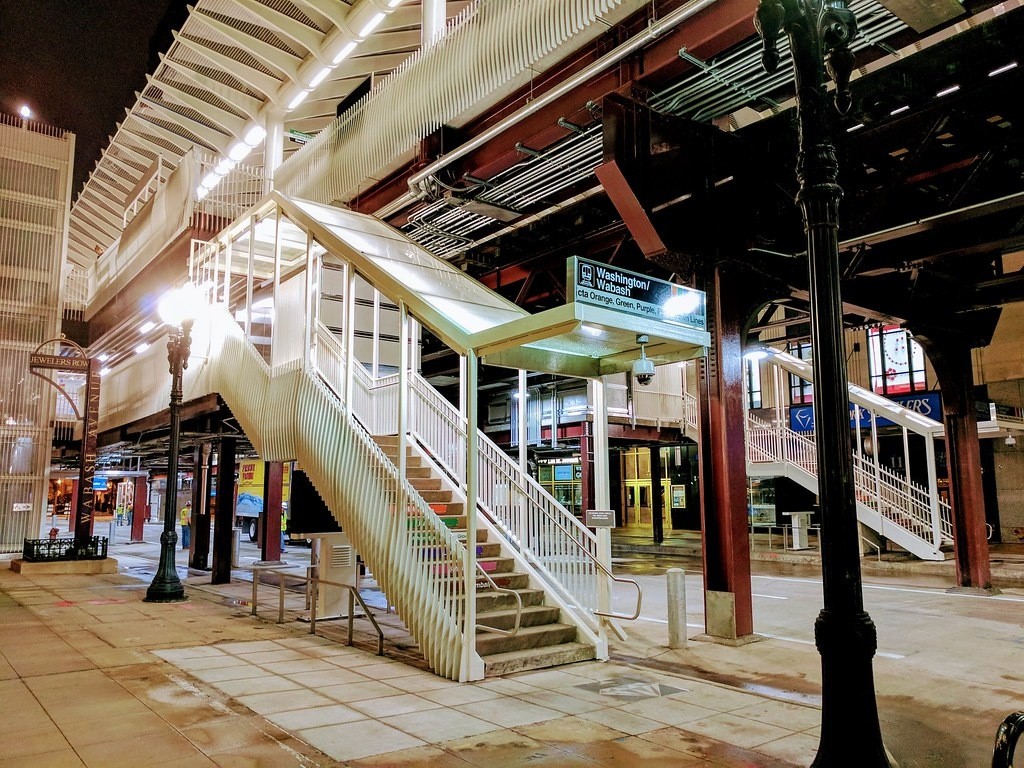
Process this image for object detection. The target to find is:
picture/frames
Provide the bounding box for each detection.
[670,485,686,509]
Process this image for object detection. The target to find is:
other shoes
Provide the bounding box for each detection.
[183,545,190,549]
[280,549,288,554]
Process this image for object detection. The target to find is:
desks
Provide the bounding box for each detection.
[781,511,816,550]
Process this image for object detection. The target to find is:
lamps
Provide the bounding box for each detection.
[742,344,768,361]
[194,0,404,202]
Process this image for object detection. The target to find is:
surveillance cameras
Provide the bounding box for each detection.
[632,360,656,386]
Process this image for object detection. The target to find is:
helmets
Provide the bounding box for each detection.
[186,501,192,506]
[128,501,132,505]
[118,503,123,507]
[282,502,288,509]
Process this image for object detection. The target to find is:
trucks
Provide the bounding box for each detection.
[234,456,315,550]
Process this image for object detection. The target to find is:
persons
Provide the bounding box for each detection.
[179,501,192,550]
[281,502,287,553]
[116,503,123,526]
[125,501,133,526]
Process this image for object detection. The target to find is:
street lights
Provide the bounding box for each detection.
[145,286,202,603]
